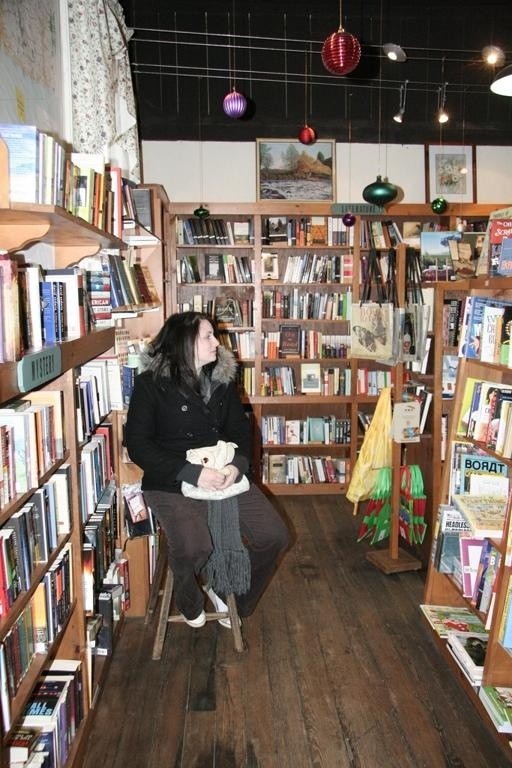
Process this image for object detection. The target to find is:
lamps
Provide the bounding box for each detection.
[392,82,408,123]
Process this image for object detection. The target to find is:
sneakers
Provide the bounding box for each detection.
[178,604,207,629]
[203,582,242,630]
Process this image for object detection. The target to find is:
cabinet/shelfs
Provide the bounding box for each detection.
[349,204,512,768]
[2,181,168,766]
[162,201,353,496]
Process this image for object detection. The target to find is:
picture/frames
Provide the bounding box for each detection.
[255,137,337,202]
[424,140,477,202]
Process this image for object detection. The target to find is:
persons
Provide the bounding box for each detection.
[126,313,290,631]
[126,311,290,629]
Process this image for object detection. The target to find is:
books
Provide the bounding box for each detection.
[175,216,255,328]
[261,449,351,484]
[4,337,152,768]
[261,286,352,359]
[261,361,352,446]
[4,252,160,362]
[261,216,354,284]
[4,126,152,241]
[359,219,418,365]
[215,331,255,434]
[420,207,510,733]
[358,362,417,444]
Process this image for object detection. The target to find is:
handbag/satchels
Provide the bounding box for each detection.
[345,386,394,516]
[351,247,430,365]
[181,439,250,501]
[356,464,429,549]
